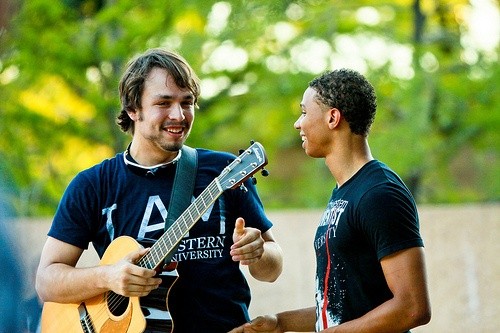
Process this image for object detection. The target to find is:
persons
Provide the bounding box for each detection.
[36,47,283,333]
[225,67,432,333]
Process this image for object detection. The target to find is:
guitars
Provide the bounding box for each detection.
[40,139,269,333]
